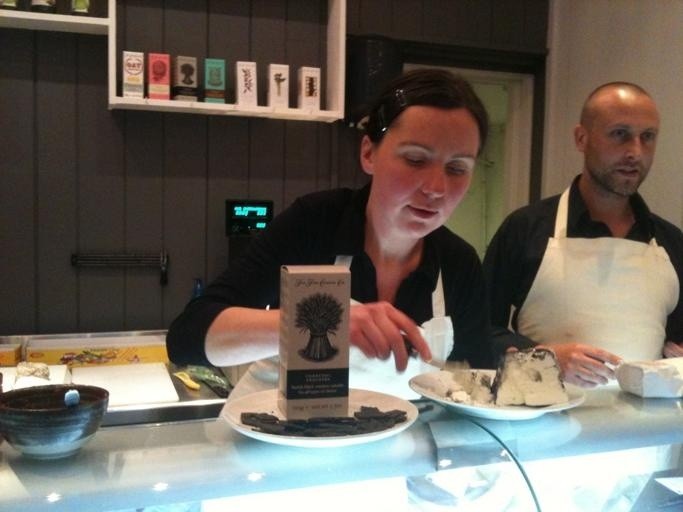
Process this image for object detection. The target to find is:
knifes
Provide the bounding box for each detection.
[165,361,227,396]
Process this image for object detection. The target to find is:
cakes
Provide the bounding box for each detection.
[439,347,567,405]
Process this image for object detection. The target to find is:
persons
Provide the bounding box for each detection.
[165,68,483,368]
[455,80,683,391]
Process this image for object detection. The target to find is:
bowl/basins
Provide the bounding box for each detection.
[1,382,110,461]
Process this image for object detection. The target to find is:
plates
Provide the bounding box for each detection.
[220,386,420,450]
[406,367,587,422]
